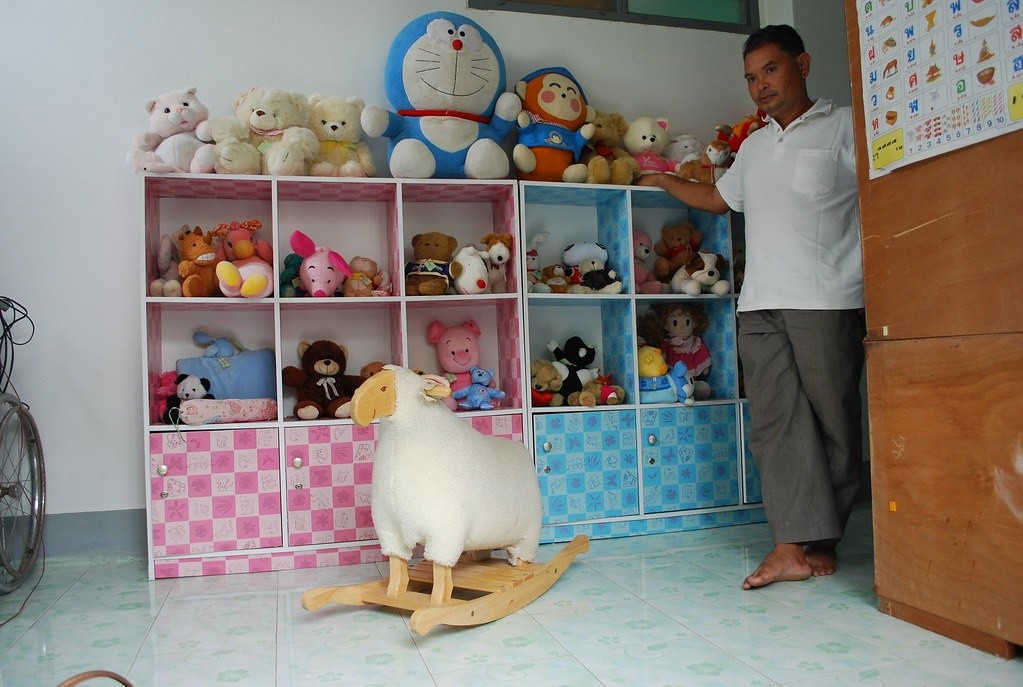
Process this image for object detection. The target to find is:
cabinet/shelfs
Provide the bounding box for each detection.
[139,168,772,584]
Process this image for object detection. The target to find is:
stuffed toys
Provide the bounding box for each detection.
[282,339,389,420]
[405,232,514,295]
[511,65,767,184]
[530,335,625,407]
[307,92,377,178]
[126,85,217,173]
[279,229,394,298]
[636,301,713,406]
[525,231,623,294]
[211,85,319,175]
[631,222,731,295]
[413,319,507,410]
[149,220,274,299]
[154,332,276,424]
[360,11,523,179]
[335,363,542,564]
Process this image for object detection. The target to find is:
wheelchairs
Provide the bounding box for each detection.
[0,296,46,594]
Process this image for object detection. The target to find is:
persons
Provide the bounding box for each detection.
[636,26,865,590]
